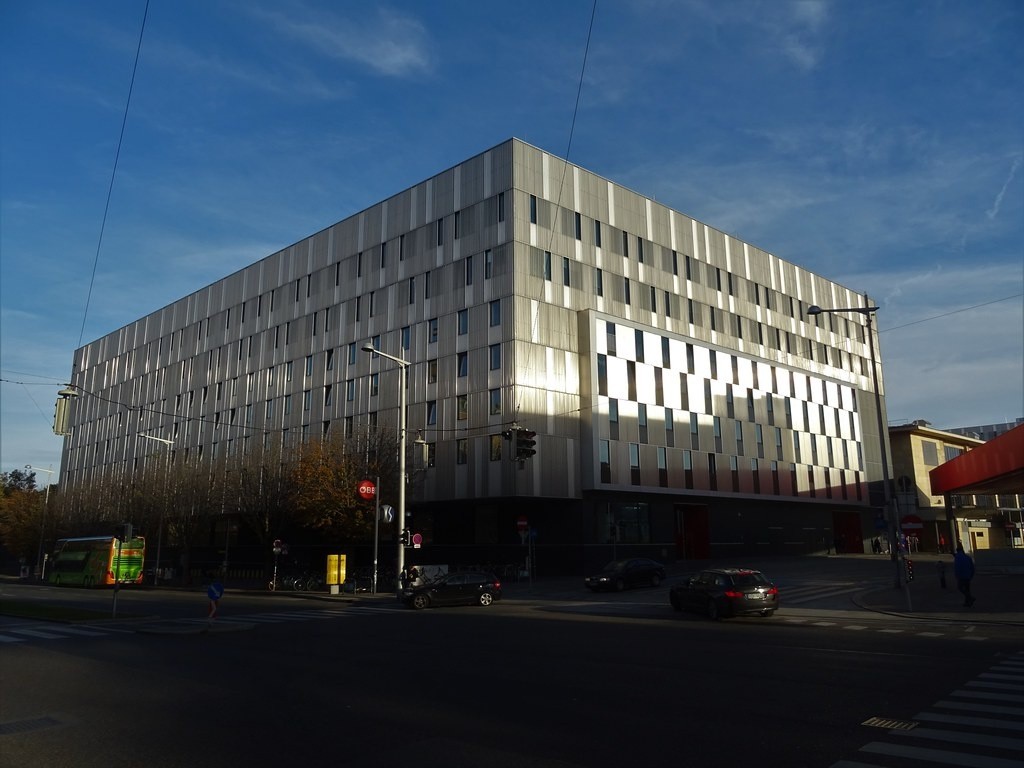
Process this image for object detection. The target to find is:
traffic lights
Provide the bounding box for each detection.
[616,520,624,542]
[40,551,44,560]
[114,523,127,543]
[512,426,537,459]
[904,558,914,584]
[44,553,48,561]
[500,429,513,441]
[413,440,436,472]
[402,530,411,546]
[609,525,617,536]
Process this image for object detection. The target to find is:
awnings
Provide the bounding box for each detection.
[154,566,174,586]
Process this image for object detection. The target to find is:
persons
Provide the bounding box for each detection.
[954,546,976,607]
[403,562,419,588]
[939,534,944,553]
[874,537,882,552]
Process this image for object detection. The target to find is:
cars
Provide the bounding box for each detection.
[400,570,504,611]
[668,568,780,621]
[585,555,669,592]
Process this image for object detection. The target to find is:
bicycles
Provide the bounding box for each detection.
[448,559,524,582]
[263,569,397,593]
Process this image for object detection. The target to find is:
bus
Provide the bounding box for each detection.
[44,534,145,589]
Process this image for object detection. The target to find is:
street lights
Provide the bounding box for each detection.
[360,341,412,600]
[24,463,54,584]
[804,294,912,612]
[137,429,176,586]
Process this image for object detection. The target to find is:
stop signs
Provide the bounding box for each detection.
[517,515,528,529]
[901,514,922,538]
[1004,521,1016,531]
